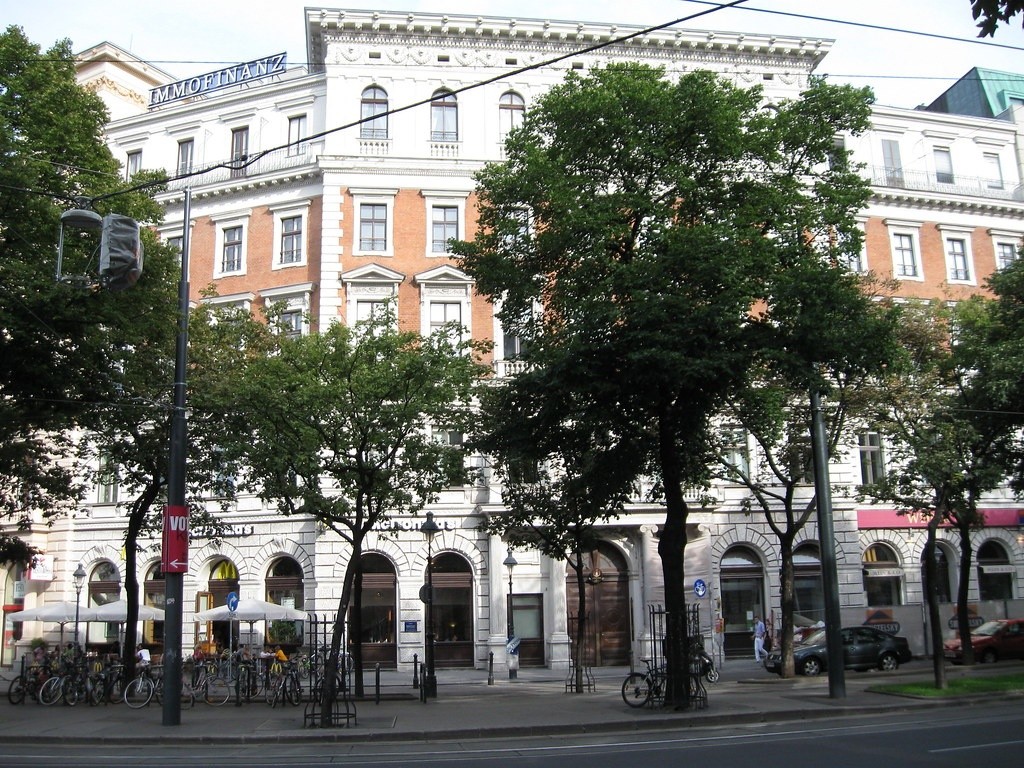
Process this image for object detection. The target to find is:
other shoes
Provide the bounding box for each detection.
[755,661,762,664]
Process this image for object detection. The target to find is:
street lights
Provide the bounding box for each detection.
[419,512,440,699]
[502,551,518,679]
[72,561,86,650]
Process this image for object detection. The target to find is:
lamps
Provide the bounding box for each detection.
[374,12,380,20]
[738,34,744,42]
[572,62,584,69]
[763,73,774,81]
[438,55,451,62]
[544,21,550,28]
[477,17,483,25]
[815,40,822,47]
[510,19,517,27]
[408,14,415,21]
[910,527,914,538]
[340,11,346,18]
[505,58,518,66]
[321,9,328,17]
[578,23,584,31]
[368,52,382,59]
[769,36,776,44]
[611,25,618,33]
[800,39,806,47]
[676,30,683,37]
[708,32,716,40]
[442,15,449,23]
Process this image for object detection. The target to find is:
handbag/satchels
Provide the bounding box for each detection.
[763,631,772,653]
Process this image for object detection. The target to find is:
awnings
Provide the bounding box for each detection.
[864,567,904,577]
[980,564,1016,574]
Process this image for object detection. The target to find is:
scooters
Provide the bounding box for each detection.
[690,642,720,683]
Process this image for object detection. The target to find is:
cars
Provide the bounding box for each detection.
[942,617,1024,665]
[762,624,913,678]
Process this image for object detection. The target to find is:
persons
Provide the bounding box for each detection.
[259,646,288,662]
[194,643,231,664]
[108,640,151,668]
[750,616,769,663]
[235,643,257,671]
[30,643,74,674]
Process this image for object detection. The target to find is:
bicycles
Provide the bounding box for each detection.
[621,659,704,709]
[5,643,352,707]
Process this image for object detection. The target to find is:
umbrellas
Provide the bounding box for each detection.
[6,600,88,665]
[64,598,165,660]
[192,598,307,664]
